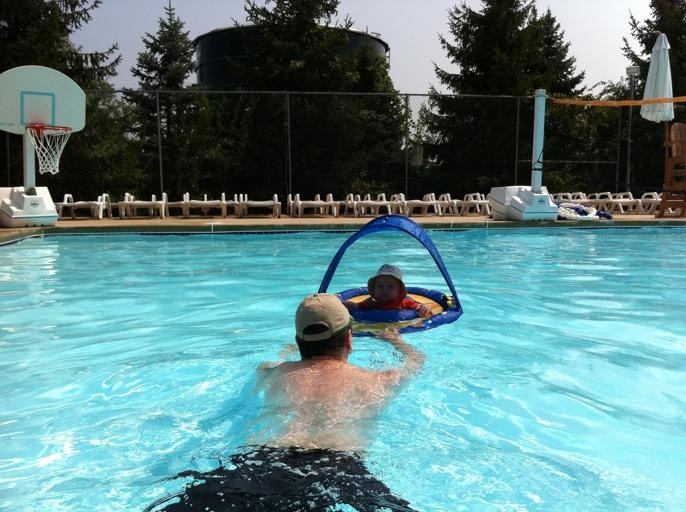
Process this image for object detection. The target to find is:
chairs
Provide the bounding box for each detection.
[56,193,681,219]
[655,123,686,218]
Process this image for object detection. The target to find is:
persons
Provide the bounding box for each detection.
[345,262,436,321]
[169,291,430,511]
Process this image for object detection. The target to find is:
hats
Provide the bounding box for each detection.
[294,293,351,342]
[367,263,408,301]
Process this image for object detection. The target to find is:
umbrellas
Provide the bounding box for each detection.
[639,31,677,124]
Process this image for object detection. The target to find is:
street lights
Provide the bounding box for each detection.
[626,66,641,192]
